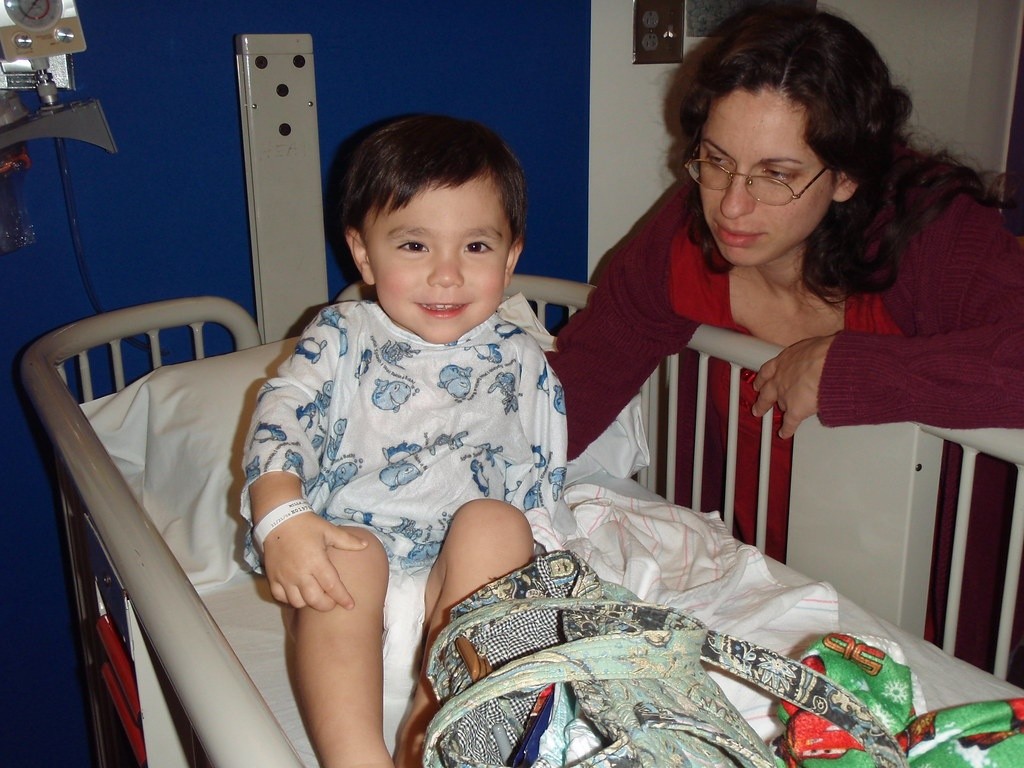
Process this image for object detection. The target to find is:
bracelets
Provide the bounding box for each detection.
[253,499,316,551]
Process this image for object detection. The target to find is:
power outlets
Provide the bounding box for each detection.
[631,0,685,64]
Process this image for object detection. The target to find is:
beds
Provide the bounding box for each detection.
[0,273,1024,768]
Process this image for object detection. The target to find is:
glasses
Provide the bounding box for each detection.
[684,142,829,207]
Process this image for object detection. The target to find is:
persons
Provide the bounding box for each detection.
[544,10,1024,676]
[242,112,568,768]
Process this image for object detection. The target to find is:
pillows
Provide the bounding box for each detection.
[78,335,300,593]
